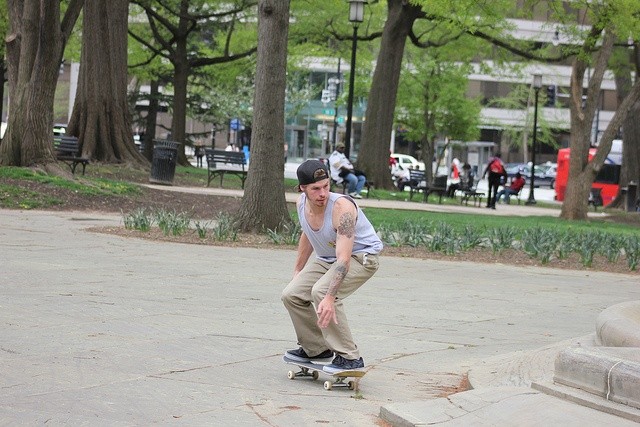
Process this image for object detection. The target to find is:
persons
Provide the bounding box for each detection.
[495,171,526,204]
[482,153,509,208]
[281,158,385,373]
[448,161,473,198]
[327,142,368,198]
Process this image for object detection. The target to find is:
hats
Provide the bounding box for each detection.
[336,143,345,147]
[297,160,329,191]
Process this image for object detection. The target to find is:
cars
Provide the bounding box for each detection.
[390,152,426,182]
[500,161,539,187]
[527,161,557,189]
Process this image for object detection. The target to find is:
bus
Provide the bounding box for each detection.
[554,142,622,207]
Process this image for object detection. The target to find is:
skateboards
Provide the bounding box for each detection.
[283,355,366,390]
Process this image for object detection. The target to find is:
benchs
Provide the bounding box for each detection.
[204,148,249,188]
[504,184,523,204]
[320,156,376,198]
[408,167,447,201]
[458,174,485,207]
[52,135,89,176]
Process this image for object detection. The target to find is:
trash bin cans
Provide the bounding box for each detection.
[149,145,178,186]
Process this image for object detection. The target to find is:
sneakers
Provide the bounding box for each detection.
[285,346,332,363]
[350,192,363,199]
[322,355,364,374]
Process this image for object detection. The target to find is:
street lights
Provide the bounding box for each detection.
[345,0,368,158]
[525,69,543,204]
